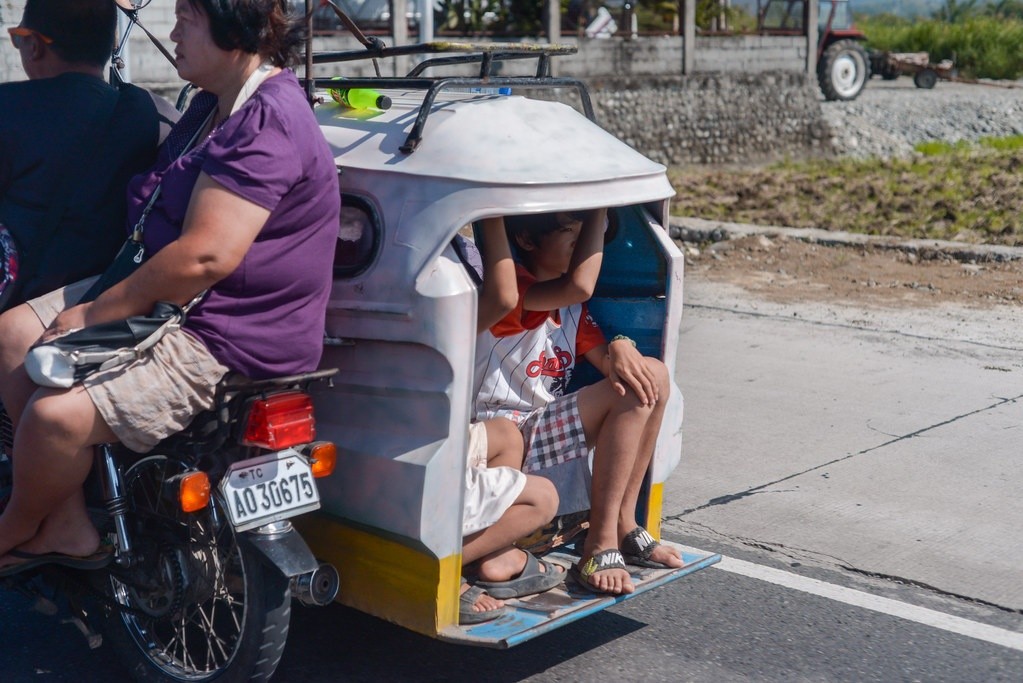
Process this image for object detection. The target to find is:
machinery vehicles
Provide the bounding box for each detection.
[759,1,953,103]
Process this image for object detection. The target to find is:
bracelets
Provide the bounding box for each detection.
[612,335,636,348]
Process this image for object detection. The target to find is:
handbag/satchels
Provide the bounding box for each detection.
[49,238,195,347]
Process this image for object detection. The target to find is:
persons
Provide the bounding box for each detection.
[0,0,336,576]
[458,207,685,625]
[0,0,160,313]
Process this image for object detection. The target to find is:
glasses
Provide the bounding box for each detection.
[7,26,52,49]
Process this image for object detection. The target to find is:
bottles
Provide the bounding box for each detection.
[434,76,515,96]
[325,76,392,111]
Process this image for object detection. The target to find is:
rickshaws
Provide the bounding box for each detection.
[0,1,724,682]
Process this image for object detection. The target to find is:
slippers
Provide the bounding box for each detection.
[0,538,116,578]
[619,526,669,569]
[570,548,628,595]
[459,576,505,624]
[475,547,567,598]
